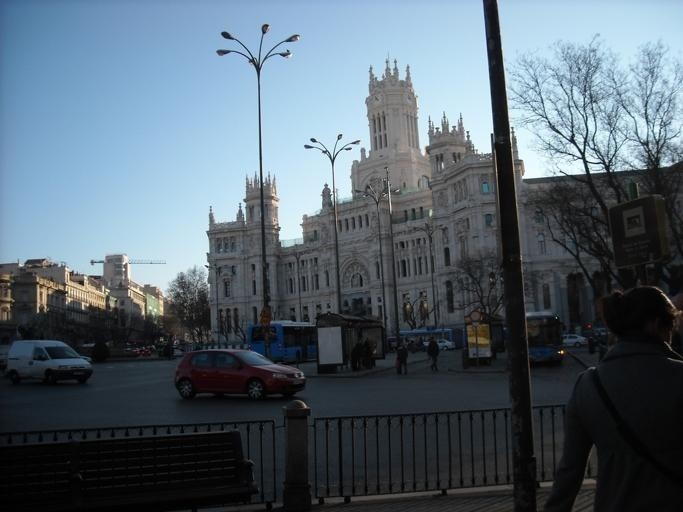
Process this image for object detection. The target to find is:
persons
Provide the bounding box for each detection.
[395,344,409,375]
[540,284,682,511]
[348,336,376,371]
[425,336,440,372]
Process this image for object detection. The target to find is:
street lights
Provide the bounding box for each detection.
[302,133,361,312]
[354,183,400,336]
[412,222,448,325]
[217,24,300,358]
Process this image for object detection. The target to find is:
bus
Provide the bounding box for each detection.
[245,318,318,362]
[525,310,565,367]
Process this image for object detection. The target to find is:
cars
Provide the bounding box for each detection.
[385,326,463,353]
[125,337,169,357]
[173,349,306,401]
[561,326,614,354]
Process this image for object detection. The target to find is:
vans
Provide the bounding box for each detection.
[0,339,95,386]
